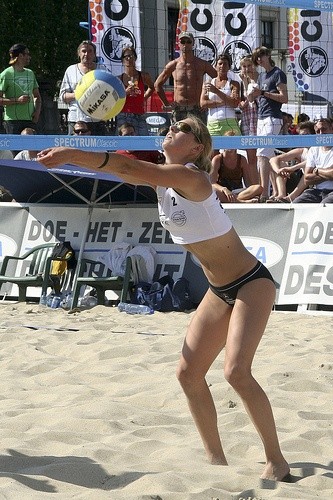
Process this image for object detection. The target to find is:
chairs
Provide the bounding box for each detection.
[70,245,151,310]
[0,243,74,306]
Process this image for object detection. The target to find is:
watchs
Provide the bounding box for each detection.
[314,168,318,174]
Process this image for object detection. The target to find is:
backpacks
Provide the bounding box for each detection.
[149,275,175,310]
[131,281,152,305]
[157,277,192,312]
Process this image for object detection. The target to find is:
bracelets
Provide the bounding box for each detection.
[96,152,109,169]
[260,91,265,96]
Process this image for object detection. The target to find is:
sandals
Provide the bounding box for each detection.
[279,196,292,202]
[266,194,277,202]
[275,196,283,201]
[259,195,268,202]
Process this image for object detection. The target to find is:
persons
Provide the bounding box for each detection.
[156,128,169,164]
[115,124,157,164]
[36,116,290,481]
[209,131,263,203]
[115,46,155,136]
[269,110,333,203]
[61,41,108,137]
[0,43,41,158]
[200,54,242,136]
[13,128,43,161]
[237,56,258,136]
[154,31,218,127]
[73,121,90,136]
[248,46,288,202]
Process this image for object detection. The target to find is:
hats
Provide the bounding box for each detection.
[179,32,194,42]
[9,44,27,64]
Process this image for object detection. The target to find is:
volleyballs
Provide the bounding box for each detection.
[75,69,128,122]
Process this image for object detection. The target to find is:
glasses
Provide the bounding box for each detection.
[255,52,268,60]
[124,132,135,136]
[172,122,202,144]
[179,40,192,44]
[316,126,331,133]
[240,53,252,58]
[74,129,90,133]
[123,55,136,59]
